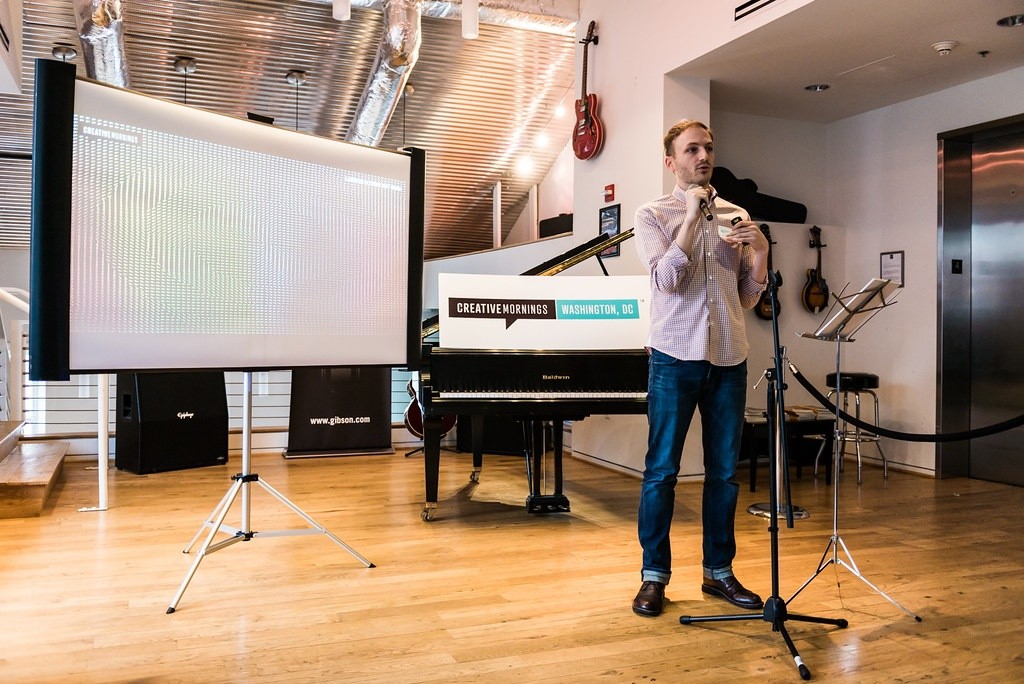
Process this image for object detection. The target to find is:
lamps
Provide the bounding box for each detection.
[332,0,351,20]
[462,0,479,39]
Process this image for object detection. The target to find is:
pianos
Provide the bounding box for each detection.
[411,228,649,522]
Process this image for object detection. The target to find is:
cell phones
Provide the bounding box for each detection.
[731,216,750,246]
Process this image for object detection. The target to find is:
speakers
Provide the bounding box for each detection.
[115,372,227,476]
[456,416,552,458]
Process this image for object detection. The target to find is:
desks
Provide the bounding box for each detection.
[743,419,837,493]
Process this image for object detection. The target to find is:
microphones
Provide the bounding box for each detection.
[687,184,713,221]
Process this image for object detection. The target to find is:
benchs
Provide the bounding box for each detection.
[0,420,73,520]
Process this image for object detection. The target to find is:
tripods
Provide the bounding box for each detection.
[679,269,848,680]
[785,279,921,622]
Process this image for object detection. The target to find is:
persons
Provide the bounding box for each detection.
[632,122,768,618]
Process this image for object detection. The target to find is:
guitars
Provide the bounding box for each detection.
[404,339,457,439]
[572,20,603,161]
[801,225,829,313]
[760,223,782,321]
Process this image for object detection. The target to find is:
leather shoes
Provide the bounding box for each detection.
[702,576,764,609]
[633,582,665,616]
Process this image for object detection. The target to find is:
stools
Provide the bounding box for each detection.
[814,372,887,484]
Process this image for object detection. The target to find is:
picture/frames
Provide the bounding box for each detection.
[598,202,621,258]
